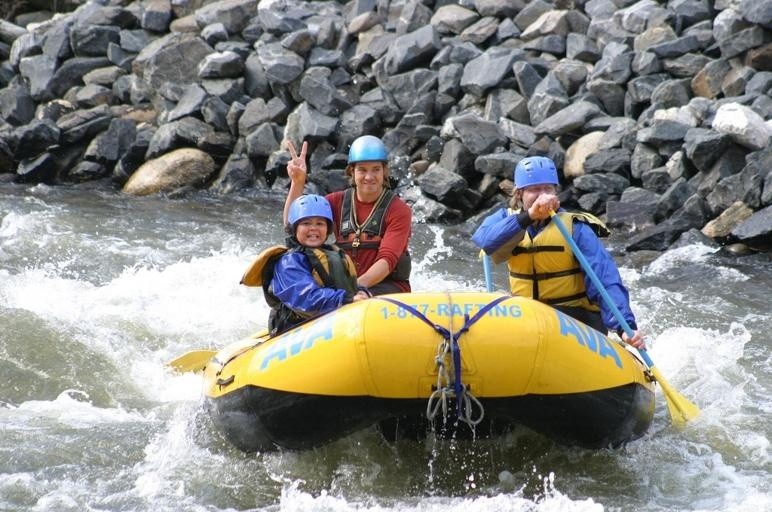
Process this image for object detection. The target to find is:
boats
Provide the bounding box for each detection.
[206,291,656,452]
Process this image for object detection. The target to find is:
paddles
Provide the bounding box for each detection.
[170,332,269,373]
[538,205,699,424]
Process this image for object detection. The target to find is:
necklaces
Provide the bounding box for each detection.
[352,187,385,247]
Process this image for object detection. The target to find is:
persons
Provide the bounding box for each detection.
[283,134,411,296]
[469,156,648,352]
[239,193,372,338]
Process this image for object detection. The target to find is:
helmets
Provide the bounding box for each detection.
[346,133,391,165]
[513,155,561,192]
[286,192,335,235]
